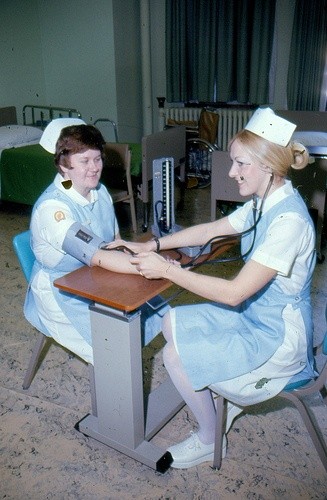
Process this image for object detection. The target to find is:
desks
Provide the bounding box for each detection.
[53,226,240,474]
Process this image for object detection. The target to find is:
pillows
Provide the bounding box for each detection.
[293,131,327,146]
[0,124,44,152]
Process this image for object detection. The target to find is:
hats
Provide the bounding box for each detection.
[39,118,87,155]
[243,106,298,148]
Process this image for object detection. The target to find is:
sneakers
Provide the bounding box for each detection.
[224,400,245,434]
[166,430,227,469]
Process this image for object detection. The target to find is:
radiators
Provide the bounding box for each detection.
[157,108,255,179]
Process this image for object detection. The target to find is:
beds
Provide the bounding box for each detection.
[0,106,187,233]
[211,110,327,264]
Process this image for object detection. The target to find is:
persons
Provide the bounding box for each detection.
[21,117,181,369]
[107,107,316,470]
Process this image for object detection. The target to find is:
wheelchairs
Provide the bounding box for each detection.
[162,104,223,191]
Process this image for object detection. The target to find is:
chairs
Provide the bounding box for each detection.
[207,359,327,472]
[101,143,137,233]
[12,230,97,416]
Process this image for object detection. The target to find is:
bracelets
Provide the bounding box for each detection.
[154,238,161,254]
